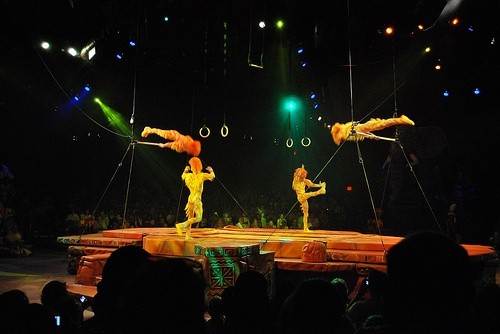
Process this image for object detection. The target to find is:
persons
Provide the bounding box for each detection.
[292,167,326,232]
[5,224,31,256]
[141,126,201,156]
[331,114,415,145]
[175,157,215,240]
[0,232,500,334]
[64,195,332,230]
